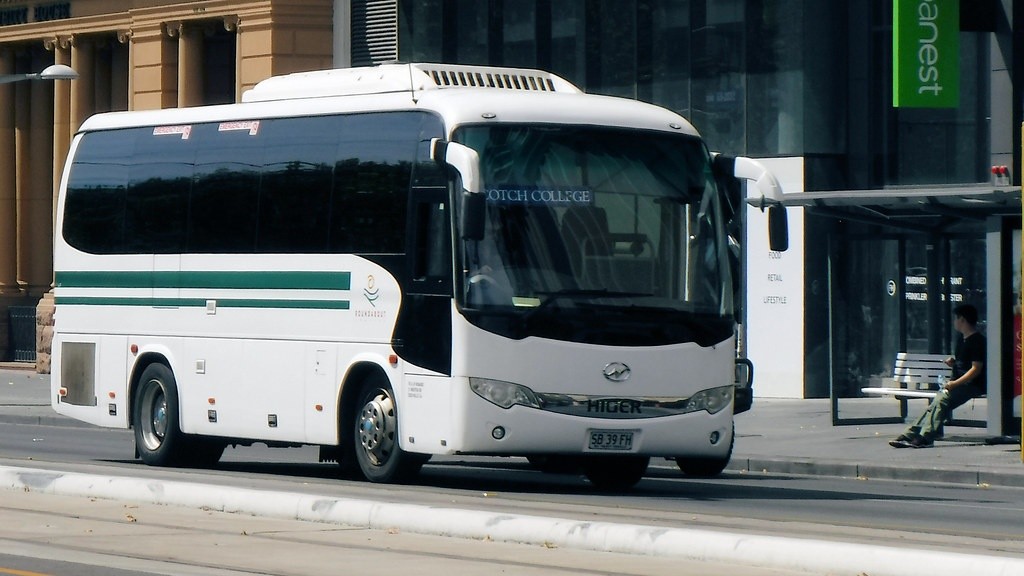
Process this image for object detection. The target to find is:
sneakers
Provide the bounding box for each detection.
[888,435,913,448]
[910,435,934,448]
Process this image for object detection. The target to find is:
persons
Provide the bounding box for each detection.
[445,239,501,275]
[888,303,987,448]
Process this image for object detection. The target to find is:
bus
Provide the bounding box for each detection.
[50,63,789,484]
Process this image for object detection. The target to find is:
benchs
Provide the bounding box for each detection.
[861,352,987,418]
